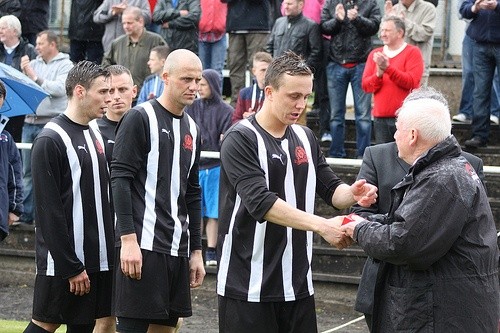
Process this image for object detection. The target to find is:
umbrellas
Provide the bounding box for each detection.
[0,62,49,118]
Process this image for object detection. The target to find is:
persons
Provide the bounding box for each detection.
[67,0,500,142]
[0,83,24,241]
[350,87,484,333]
[184,69,235,266]
[109,49,206,333]
[96,64,137,173]
[216,50,378,333]
[21,60,115,333]
[0,0,50,47]
[0,15,37,72]
[340,97,500,333]
[362,15,423,144]
[319,0,382,160]
[20,31,74,222]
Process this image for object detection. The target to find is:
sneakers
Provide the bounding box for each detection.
[205,249,218,266]
[489,113,499,125]
[452,113,472,122]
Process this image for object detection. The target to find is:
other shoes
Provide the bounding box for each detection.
[463,135,487,147]
[12,220,33,226]
[320,130,333,142]
